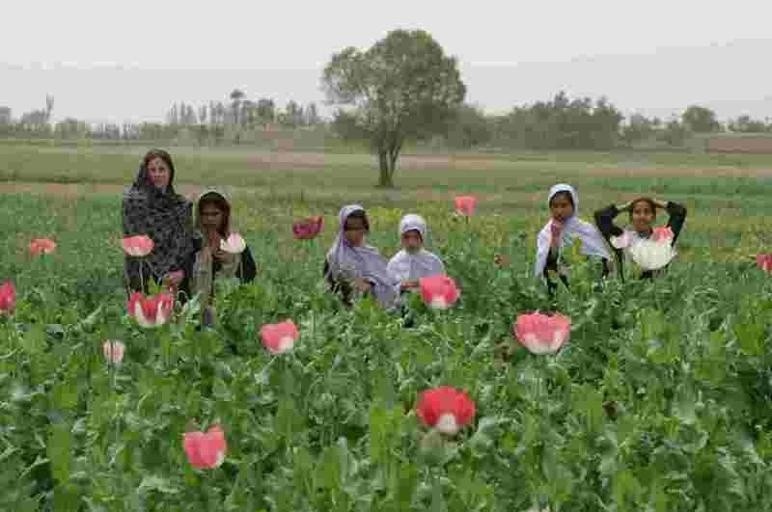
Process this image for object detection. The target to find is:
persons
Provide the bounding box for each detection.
[322,204,395,311]
[594,198,687,279]
[535,182,615,297]
[180,191,257,304]
[386,214,447,295]
[121,149,194,305]
